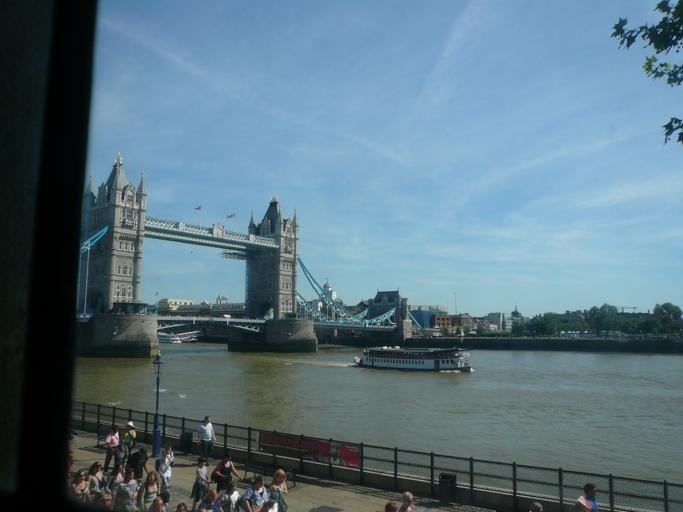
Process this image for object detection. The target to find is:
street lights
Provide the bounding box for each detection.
[149,350,166,459]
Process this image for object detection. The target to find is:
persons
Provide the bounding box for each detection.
[399,492,413,512]
[528,502,543,512]
[383,501,399,512]
[196,416,217,464]
[323,441,347,467]
[574,482,597,511]
[68,418,290,512]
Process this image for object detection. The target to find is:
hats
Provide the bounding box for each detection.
[125,420,135,429]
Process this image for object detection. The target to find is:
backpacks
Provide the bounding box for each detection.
[269,491,287,512]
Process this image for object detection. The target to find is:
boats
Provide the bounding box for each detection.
[159,330,201,343]
[354,345,472,371]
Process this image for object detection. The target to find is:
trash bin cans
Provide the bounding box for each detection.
[439,472,456,506]
[180,432,193,454]
